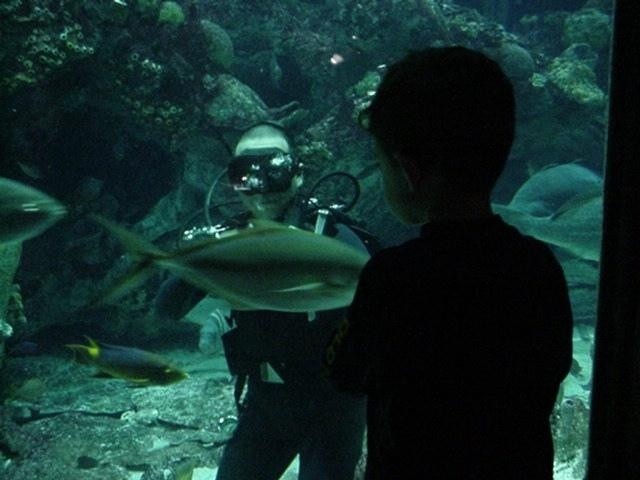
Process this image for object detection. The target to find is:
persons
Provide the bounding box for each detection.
[319,44,574,480]
[153,121,372,480]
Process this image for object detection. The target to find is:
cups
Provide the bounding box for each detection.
[224,152,294,196]
